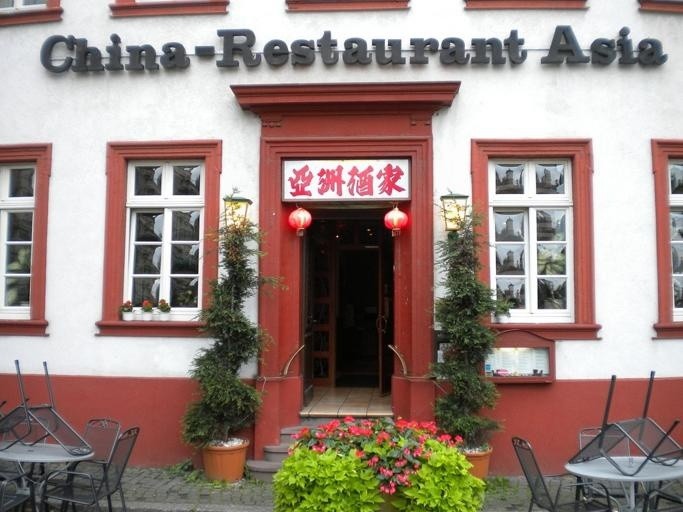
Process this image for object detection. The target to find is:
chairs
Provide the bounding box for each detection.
[0,358,140,512]
[512,369,683,512]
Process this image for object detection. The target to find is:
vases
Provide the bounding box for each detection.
[161,311,171,321]
[143,311,153,321]
[122,310,134,321]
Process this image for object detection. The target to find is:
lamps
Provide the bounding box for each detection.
[222,197,254,231]
[441,192,469,232]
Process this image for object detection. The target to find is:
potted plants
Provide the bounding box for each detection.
[431,188,505,480]
[178,187,291,485]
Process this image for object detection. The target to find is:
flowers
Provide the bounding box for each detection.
[160,299,169,312]
[121,300,134,313]
[141,300,152,311]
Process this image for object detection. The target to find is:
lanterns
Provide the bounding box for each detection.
[382,207,410,239]
[287,206,314,238]
